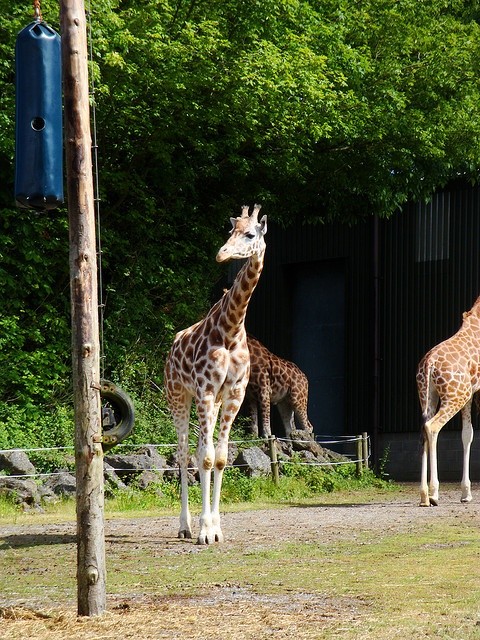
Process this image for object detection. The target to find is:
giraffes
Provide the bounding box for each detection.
[163,204,268,543]
[246,334,313,438]
[416,293,480,507]
